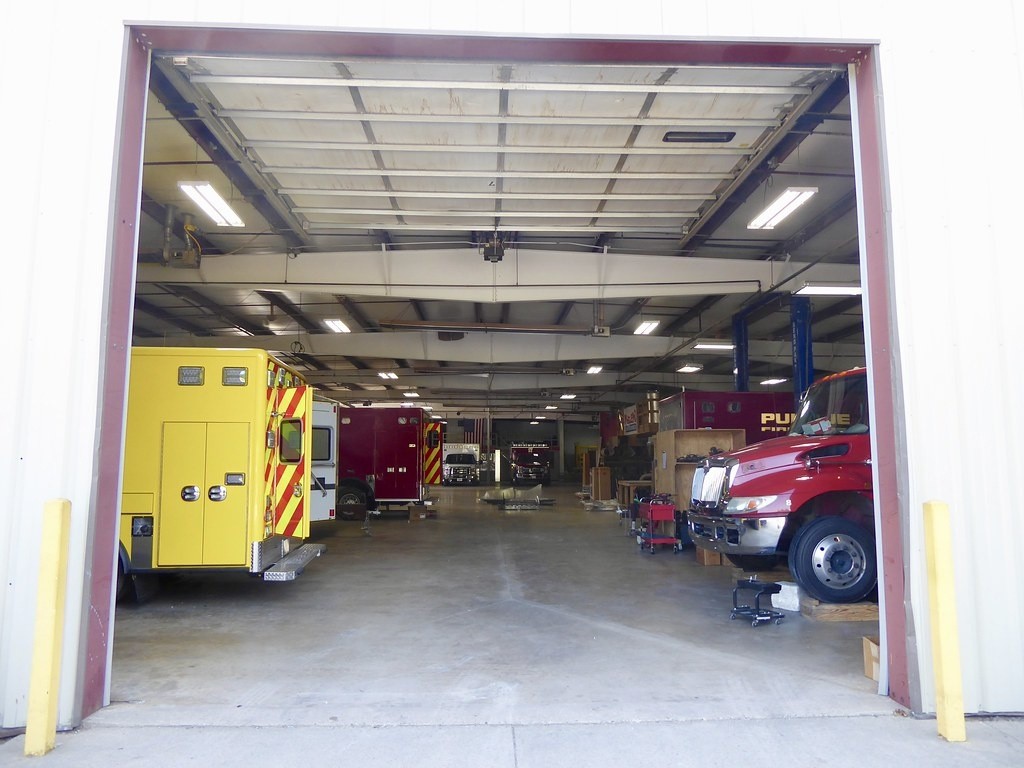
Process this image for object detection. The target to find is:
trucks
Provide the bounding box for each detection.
[311,400,336,522]
[443,443,480,485]
[116,347,328,603]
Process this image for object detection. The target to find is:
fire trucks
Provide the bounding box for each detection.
[686,366,877,605]
[339,407,443,520]
[509,442,551,486]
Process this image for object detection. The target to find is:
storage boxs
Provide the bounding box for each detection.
[695,546,722,566]
[408,505,427,521]
[862,635,880,683]
[721,552,734,566]
[771,580,800,612]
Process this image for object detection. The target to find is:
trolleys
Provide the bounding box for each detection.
[638,496,678,554]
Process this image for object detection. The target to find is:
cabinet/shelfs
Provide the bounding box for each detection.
[655,429,746,512]
